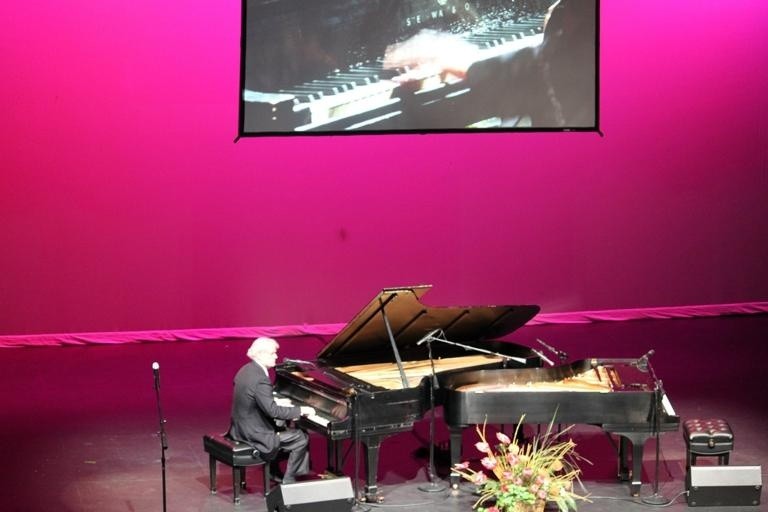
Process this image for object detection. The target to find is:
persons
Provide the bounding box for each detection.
[219,337,315,485]
[304,397,347,419]
[382,1,598,128]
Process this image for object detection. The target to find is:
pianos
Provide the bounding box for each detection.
[241,1,548,134]
[270,284,542,504]
[442,356,681,499]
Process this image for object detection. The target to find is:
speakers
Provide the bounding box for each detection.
[266,477,356,512]
[688,465,763,506]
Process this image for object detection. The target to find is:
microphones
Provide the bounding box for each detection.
[283,357,315,367]
[151,361,160,392]
[637,349,656,365]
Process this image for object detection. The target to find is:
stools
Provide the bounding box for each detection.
[683,418,734,472]
[204,432,271,505]
